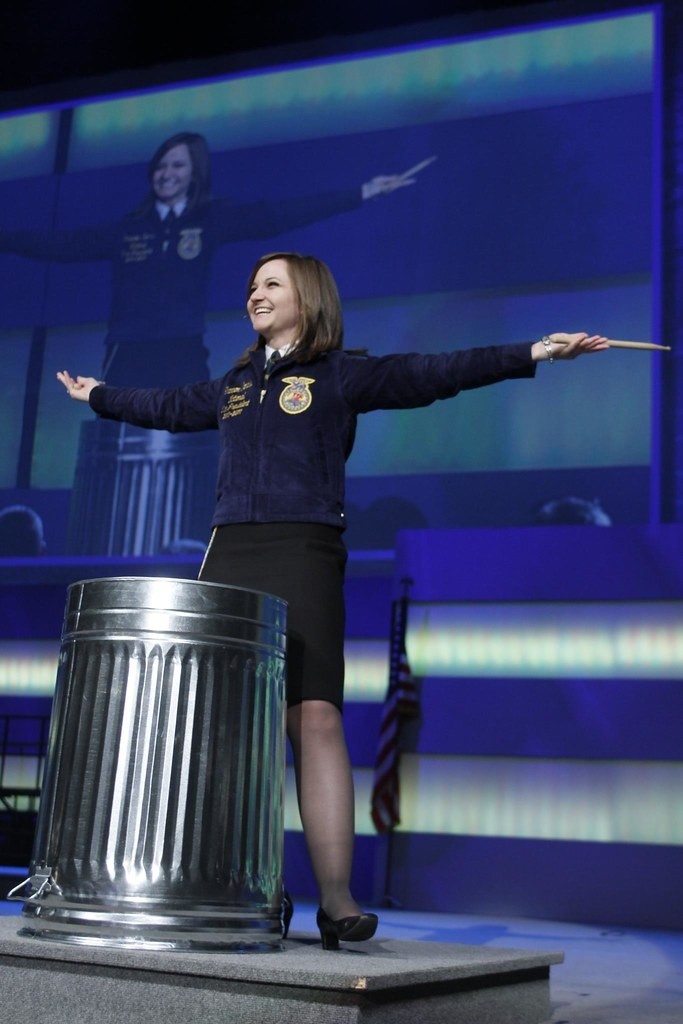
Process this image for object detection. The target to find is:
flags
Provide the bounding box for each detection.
[371,598,422,836]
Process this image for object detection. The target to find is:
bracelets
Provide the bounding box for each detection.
[541,336,555,363]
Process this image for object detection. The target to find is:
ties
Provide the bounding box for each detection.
[262,350,283,393]
[161,207,179,250]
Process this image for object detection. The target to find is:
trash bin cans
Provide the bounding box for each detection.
[4,574,291,952]
[64,418,208,559]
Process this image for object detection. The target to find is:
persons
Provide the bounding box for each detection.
[52,256,609,951]
[0,132,418,394]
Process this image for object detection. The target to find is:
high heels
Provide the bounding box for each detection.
[282,891,294,939]
[315,904,379,951]
